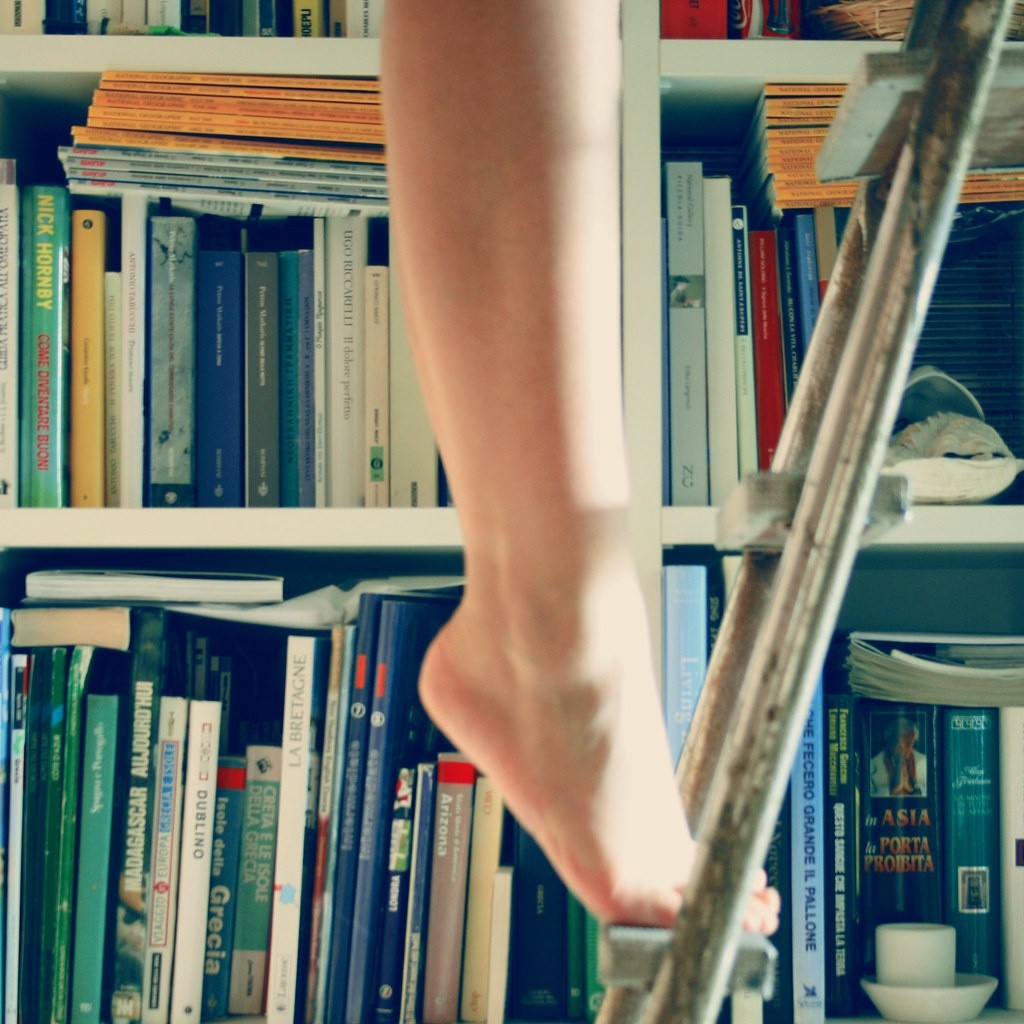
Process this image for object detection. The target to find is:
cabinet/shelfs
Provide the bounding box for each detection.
[1,0,1024,1024]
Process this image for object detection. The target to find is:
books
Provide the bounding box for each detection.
[653,80,1024,507]
[0,70,452,507]
[0,0,384,39]
[662,555,1024,1024]
[0,566,608,1024]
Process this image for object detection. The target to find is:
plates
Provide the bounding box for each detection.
[861,973,999,1022]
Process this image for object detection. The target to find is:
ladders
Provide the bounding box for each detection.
[578,1,1023,1024]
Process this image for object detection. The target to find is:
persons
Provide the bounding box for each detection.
[378,0,785,938]
[669,276,698,308]
[870,719,926,797]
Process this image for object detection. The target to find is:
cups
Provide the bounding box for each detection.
[875,923,957,990]
